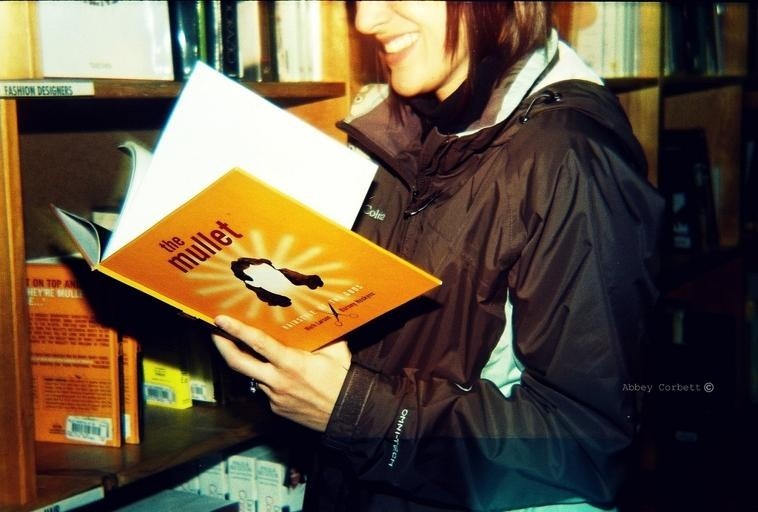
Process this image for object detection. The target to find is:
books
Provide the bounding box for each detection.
[0,1,445,512]
[569,0,729,510]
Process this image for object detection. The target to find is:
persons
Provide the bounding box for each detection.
[213,0,666,511]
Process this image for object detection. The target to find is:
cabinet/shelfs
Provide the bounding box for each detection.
[0,2,756,512]
